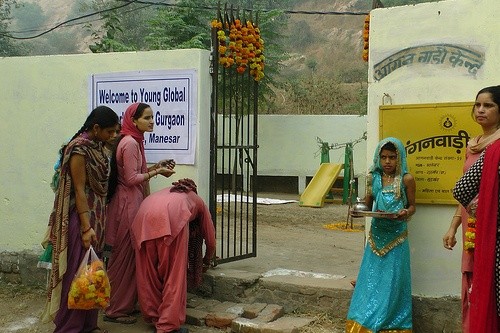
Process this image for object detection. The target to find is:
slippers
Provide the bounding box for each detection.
[103,313,137,324]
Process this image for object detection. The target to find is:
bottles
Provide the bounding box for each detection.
[355,202,368,211]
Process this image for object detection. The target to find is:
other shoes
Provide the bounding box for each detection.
[165,325,188,333]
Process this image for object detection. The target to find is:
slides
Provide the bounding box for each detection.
[298,163,345,207]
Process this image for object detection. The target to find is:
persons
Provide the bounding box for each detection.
[38,105,120,333]
[102,123,122,160]
[130,179,216,333]
[443,85,500,333]
[102,102,176,323]
[344,137,416,333]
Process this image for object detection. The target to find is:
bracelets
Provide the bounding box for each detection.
[82,225,91,234]
[454,214,462,218]
[404,209,408,218]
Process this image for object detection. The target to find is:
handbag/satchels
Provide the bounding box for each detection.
[67,243,111,309]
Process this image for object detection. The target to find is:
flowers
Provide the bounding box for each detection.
[69,261,112,310]
[210,20,266,84]
